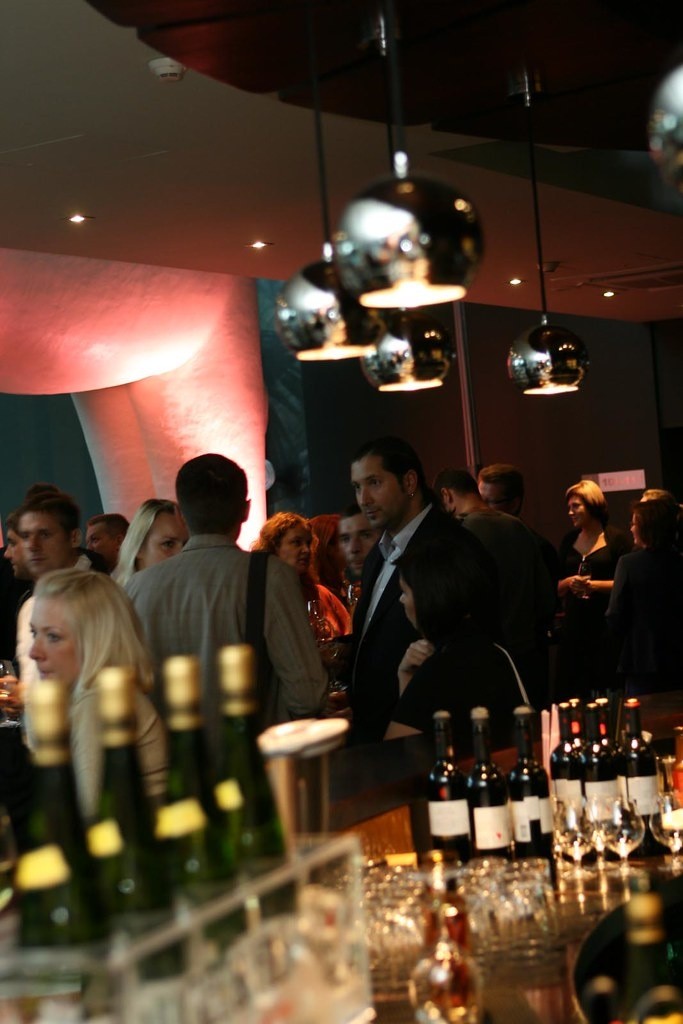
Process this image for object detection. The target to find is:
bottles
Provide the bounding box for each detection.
[85,665,182,978]
[550,698,662,829]
[218,644,296,916]
[427,711,471,863]
[505,706,555,859]
[165,656,246,948]
[18,681,107,949]
[464,706,514,863]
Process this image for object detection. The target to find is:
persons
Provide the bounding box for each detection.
[122,454,328,730]
[254,463,556,717]
[383,539,530,740]
[548,480,629,704]
[31,569,170,820]
[0,480,188,679]
[605,488,683,697]
[329,436,512,746]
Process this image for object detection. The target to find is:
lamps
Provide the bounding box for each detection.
[334,0,484,312]
[502,68,591,396]
[360,312,453,392]
[272,1,387,361]
[646,62,683,200]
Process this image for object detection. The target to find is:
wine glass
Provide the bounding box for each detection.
[649,793,683,870]
[307,599,333,640]
[599,799,645,875]
[577,563,591,599]
[0,660,22,728]
[318,637,348,691]
[553,798,597,880]
[585,842,619,870]
